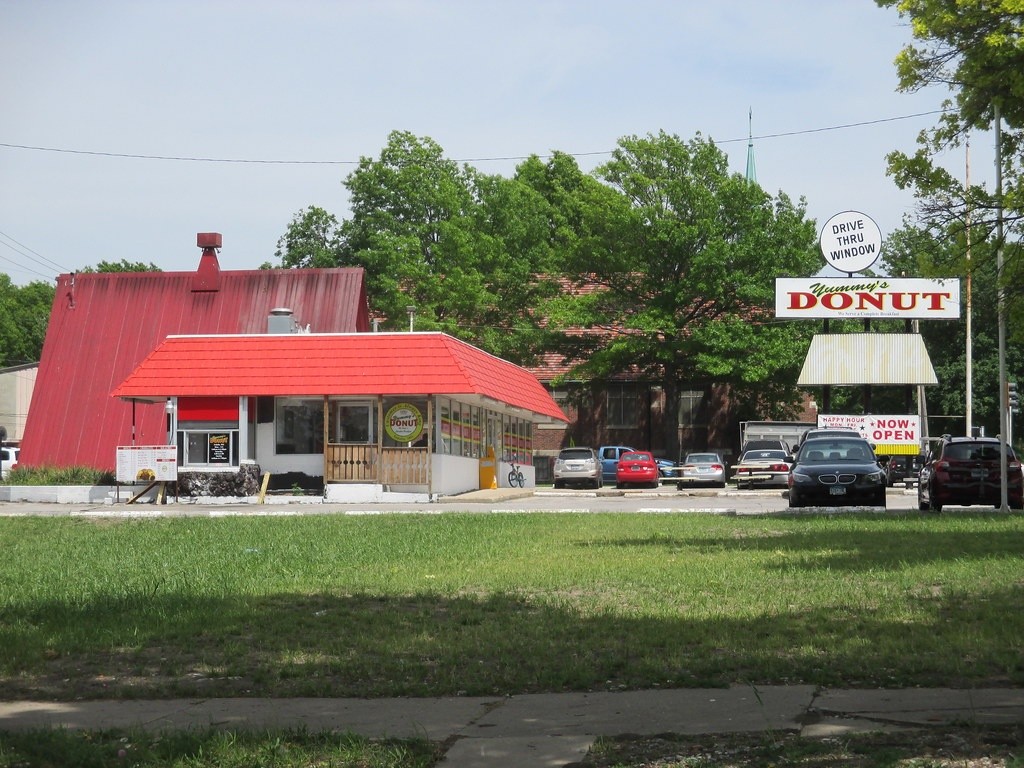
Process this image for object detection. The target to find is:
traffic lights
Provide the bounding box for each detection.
[1012,407,1021,417]
[1007,381,1019,405]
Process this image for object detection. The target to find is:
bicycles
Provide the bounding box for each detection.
[508,462,527,488]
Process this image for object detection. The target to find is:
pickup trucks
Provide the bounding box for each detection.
[596,445,677,484]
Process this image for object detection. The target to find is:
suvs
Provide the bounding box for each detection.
[791,426,863,454]
[553,446,605,490]
[917,434,1024,513]
[736,440,793,468]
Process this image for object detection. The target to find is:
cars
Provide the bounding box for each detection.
[782,437,890,508]
[885,452,923,487]
[680,452,728,489]
[0,447,21,482]
[614,452,660,491]
[734,449,792,489]
